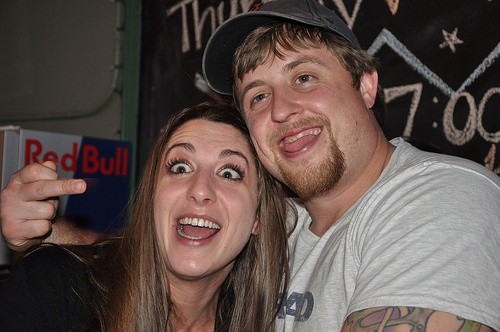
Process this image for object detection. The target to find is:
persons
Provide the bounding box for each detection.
[0,100,288,332]
[1,0,500,331]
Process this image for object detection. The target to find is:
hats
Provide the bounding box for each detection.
[203,0,361,96]
